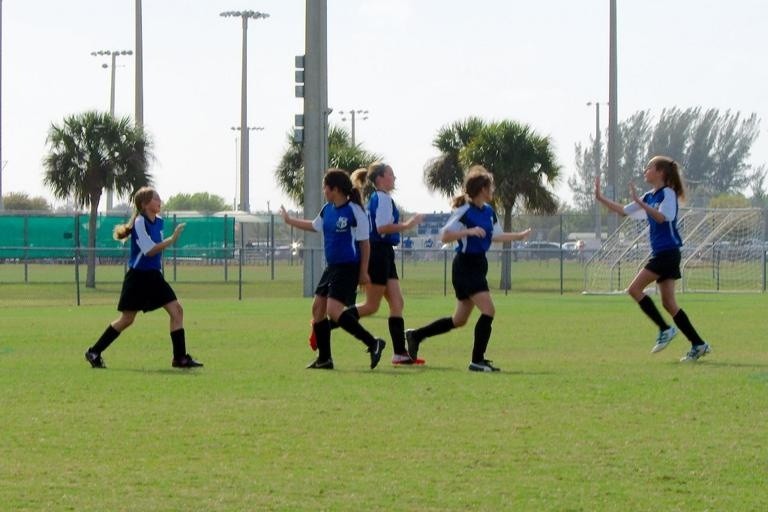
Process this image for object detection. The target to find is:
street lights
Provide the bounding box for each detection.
[230,122,266,134]
[217,8,271,216]
[335,106,370,148]
[85,47,133,212]
[583,101,609,243]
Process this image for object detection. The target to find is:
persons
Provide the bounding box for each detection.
[309,162,424,366]
[406,166,532,372]
[279,168,385,369]
[595,156,710,363]
[84,187,204,368]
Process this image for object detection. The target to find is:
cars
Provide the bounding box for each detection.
[518,238,582,260]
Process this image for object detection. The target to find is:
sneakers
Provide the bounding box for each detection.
[308,318,318,351]
[468,359,500,373]
[85,347,106,369]
[367,329,425,368]
[171,354,203,368]
[651,327,678,353]
[305,358,334,370]
[678,341,712,363]
